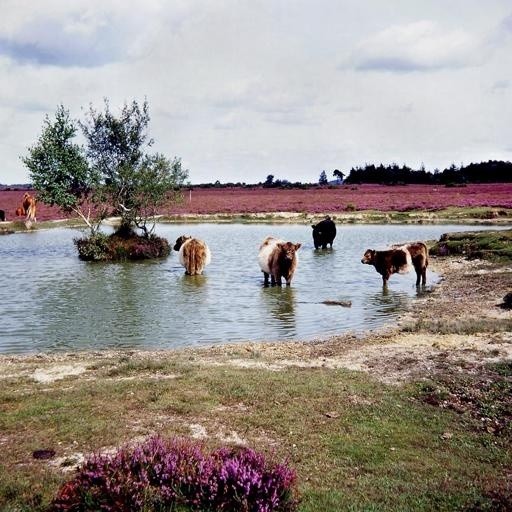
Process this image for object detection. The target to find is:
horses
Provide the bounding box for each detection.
[22,193,37,224]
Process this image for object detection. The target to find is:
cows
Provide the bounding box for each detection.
[258,236,301,288]
[173,235,211,276]
[310,218,336,251]
[361,240,429,293]
